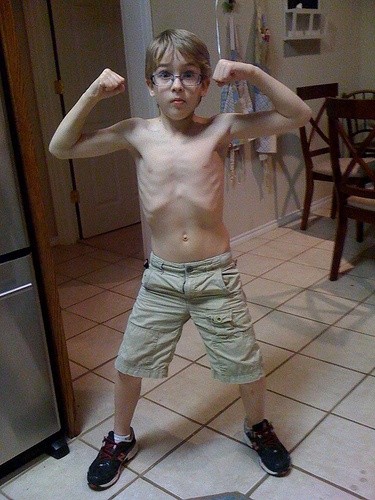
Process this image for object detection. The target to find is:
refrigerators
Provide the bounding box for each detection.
[0,87,63,466]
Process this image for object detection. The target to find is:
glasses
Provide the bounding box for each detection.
[152,73,203,88]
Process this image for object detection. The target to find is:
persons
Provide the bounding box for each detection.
[48,28,313,491]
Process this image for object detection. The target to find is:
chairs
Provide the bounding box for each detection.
[296,83,375,281]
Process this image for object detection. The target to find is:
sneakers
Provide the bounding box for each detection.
[87,427,138,487]
[243,417,291,477]
